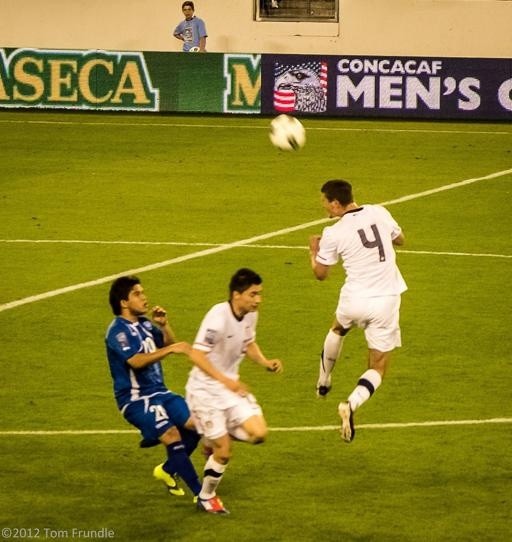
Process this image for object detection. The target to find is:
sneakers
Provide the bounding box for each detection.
[153,461,185,497]
[337,400,355,443]
[200,445,214,458]
[193,494,230,516]
[316,372,333,399]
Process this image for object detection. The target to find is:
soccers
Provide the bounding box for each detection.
[189,47,200,52]
[268,114,306,151]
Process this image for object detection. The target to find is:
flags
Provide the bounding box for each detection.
[272,60,327,113]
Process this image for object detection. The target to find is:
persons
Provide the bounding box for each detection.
[174,1,208,52]
[184,268,284,517]
[307,178,408,445]
[104,275,203,505]
